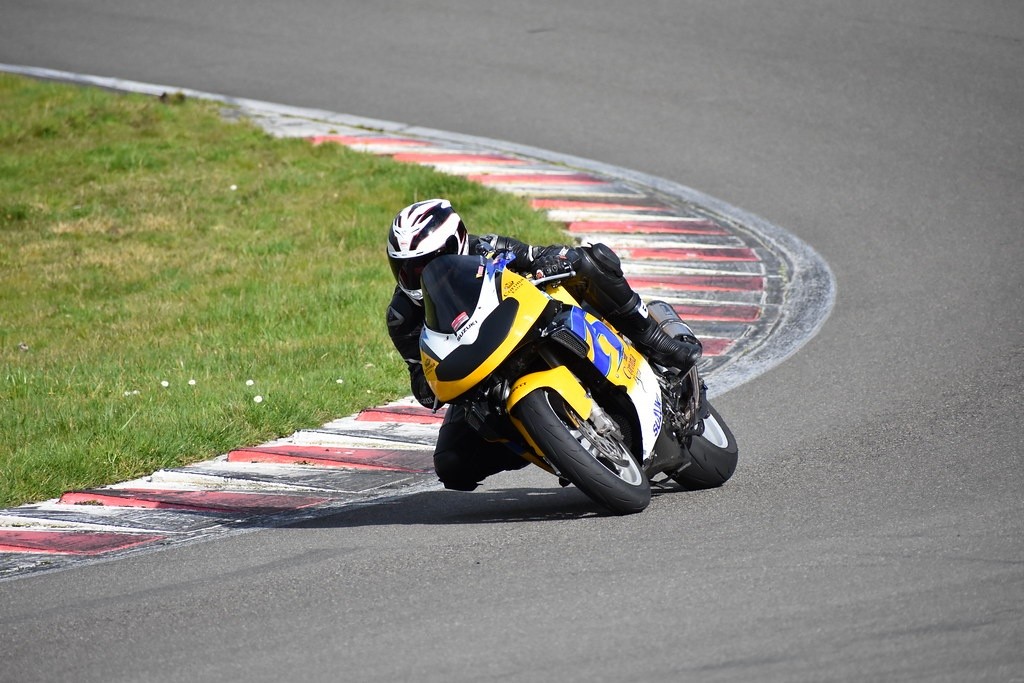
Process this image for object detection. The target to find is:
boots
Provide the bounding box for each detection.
[638,317,702,367]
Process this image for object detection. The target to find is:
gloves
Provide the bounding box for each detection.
[530,255,573,292]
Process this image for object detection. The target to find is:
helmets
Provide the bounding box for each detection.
[386,199,470,309]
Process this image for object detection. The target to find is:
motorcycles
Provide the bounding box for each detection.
[420,242,740,517]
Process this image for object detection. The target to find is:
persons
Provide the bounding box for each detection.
[386,198,701,492]
